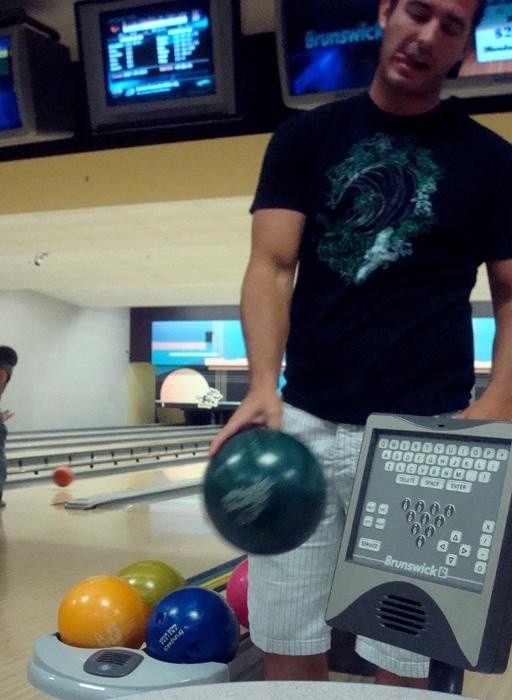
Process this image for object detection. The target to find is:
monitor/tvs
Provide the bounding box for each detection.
[471,300,499,387]
[129,306,285,426]
[277,0,384,111]
[457,0,510,114]
[0,24,70,146]
[73,0,236,130]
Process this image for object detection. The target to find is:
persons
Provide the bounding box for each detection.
[204,0,509,692]
[2,343,20,512]
